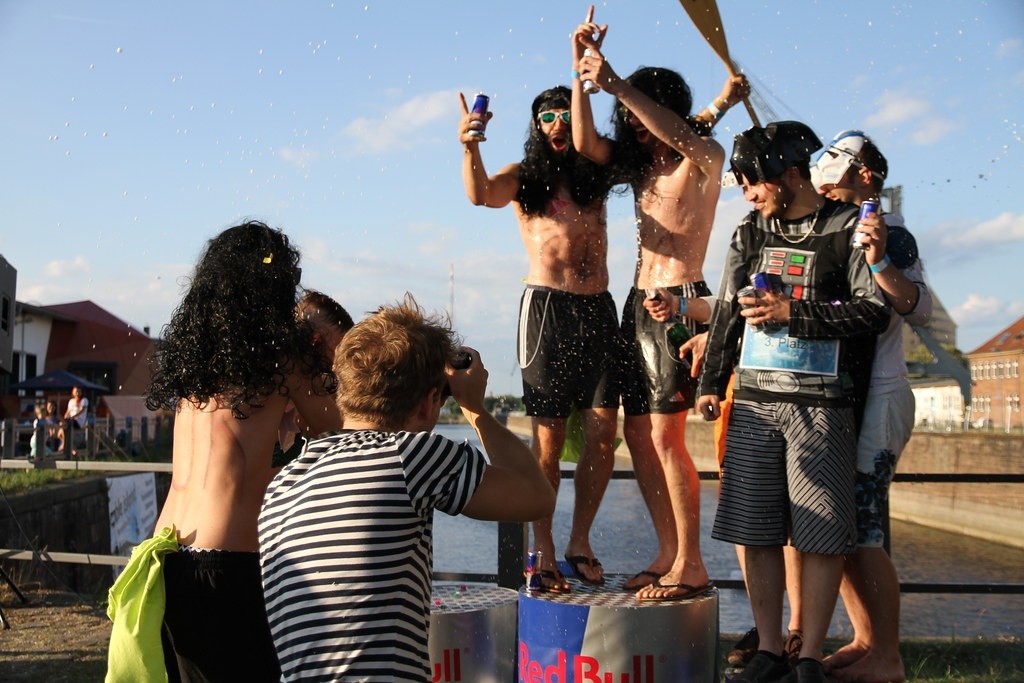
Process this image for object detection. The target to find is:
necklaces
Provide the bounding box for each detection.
[776,196,822,244]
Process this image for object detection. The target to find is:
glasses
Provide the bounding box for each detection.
[537,109,571,124]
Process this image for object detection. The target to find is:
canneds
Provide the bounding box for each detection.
[737,271,776,334]
[468,94,490,136]
[852,201,878,249]
[583,48,600,94]
[526,550,544,590]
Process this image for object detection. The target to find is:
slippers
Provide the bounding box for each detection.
[537,569,571,594]
[623,570,714,601]
[564,552,605,586]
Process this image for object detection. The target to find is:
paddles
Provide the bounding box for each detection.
[679,0,760,125]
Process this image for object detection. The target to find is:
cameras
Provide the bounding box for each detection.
[442,352,471,397]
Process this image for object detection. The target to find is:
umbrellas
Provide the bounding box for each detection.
[14,368,109,417]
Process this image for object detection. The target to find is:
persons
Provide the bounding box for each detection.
[459,60,753,594]
[134,217,556,683]
[29,385,89,457]
[571,4,727,601]
[640,120,932,683]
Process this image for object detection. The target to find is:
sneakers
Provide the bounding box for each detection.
[727,626,759,666]
[785,629,804,669]
[794,661,829,683]
[731,653,797,683]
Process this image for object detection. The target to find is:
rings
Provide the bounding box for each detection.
[656,306,660,312]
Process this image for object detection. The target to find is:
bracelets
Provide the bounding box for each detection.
[869,254,890,274]
[706,102,725,119]
[677,294,687,315]
[570,70,580,79]
